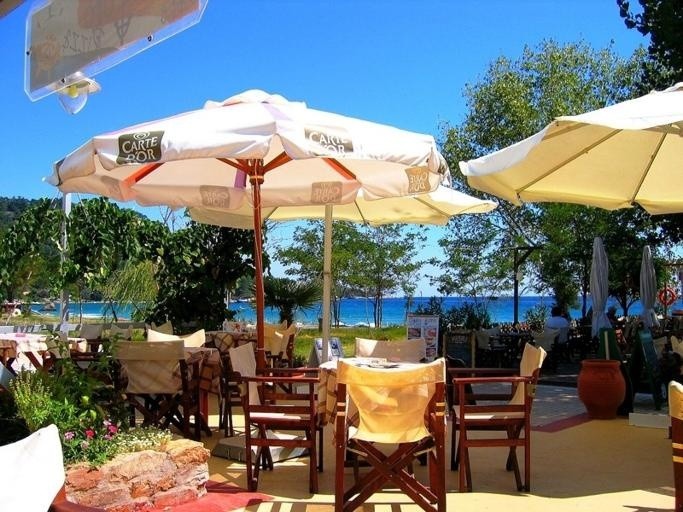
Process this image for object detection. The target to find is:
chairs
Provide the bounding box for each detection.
[668,381,683,512]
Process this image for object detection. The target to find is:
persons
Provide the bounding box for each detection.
[607,305,626,326]
[544,307,570,343]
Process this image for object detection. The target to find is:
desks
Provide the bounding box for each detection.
[0,335,86,373]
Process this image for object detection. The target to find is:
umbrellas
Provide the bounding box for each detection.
[639,244,660,329]
[41,88,450,374]
[459,82,683,216]
[187,185,497,361]
[590,236,615,360]
